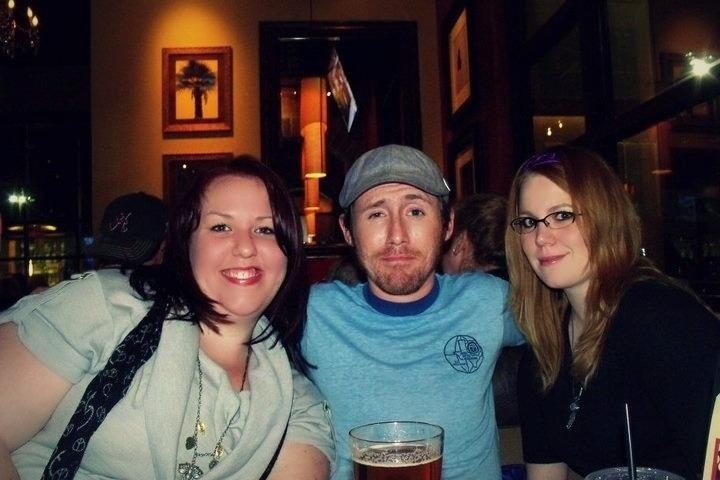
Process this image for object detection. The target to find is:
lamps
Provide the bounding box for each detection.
[279,71,328,235]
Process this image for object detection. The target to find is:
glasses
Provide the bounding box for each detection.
[511,211,582,234]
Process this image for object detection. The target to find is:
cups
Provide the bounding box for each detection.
[349,422,445,479]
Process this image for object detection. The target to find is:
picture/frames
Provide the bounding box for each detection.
[448,8,473,116]
[163,153,234,203]
[161,45,234,140]
[455,145,476,197]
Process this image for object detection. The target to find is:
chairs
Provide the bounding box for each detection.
[490,346,530,424]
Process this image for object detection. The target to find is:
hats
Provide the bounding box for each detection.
[339,145,449,209]
[98,191,167,270]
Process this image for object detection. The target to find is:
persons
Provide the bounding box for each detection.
[323,249,368,292]
[84,189,165,274]
[434,191,535,480]
[284,140,543,480]
[505,137,718,480]
[0,152,339,478]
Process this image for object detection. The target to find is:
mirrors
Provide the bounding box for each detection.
[258,18,424,258]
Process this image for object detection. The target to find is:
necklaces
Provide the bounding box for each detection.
[565,313,601,432]
[183,343,255,480]
